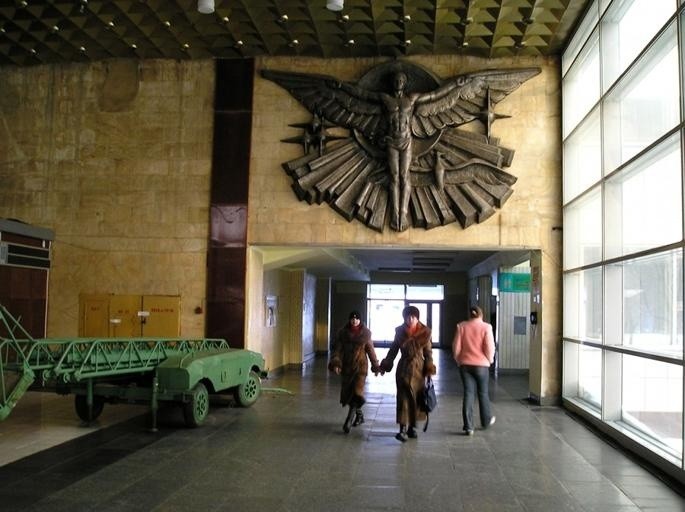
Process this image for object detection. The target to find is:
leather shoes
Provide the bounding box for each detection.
[407,431,417,438]
[396,433,408,441]
[490,416,496,424]
[467,429,474,436]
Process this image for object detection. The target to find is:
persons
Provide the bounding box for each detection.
[326,68,469,232]
[380,305,437,442]
[328,311,379,434]
[451,306,497,435]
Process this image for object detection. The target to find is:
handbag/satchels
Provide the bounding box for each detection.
[419,375,436,412]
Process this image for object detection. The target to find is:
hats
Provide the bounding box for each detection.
[350,311,361,319]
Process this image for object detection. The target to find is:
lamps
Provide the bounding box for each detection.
[326,0,345,11]
[197,0,216,15]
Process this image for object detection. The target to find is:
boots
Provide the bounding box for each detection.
[343,419,351,433]
[353,415,365,426]
